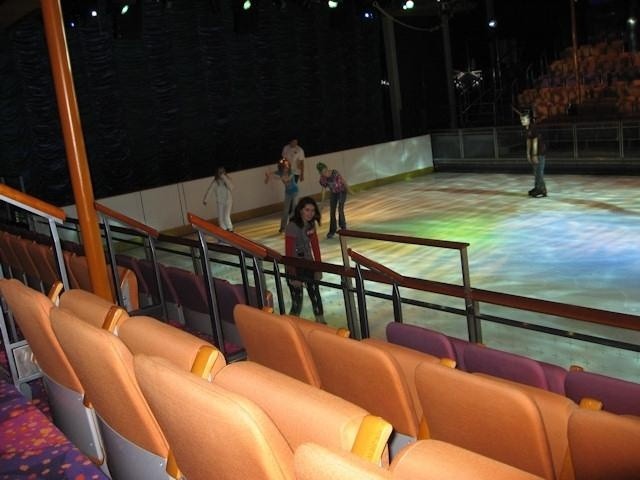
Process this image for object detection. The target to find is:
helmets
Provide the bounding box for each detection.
[277,159,290,169]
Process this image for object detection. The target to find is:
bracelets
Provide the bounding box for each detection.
[531,154,538,156]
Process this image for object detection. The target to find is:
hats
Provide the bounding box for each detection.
[316,162,327,172]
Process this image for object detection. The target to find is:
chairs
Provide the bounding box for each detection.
[0,219,640,480]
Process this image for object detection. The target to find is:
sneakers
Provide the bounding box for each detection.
[279,227,284,232]
[326,232,335,238]
[529,188,546,195]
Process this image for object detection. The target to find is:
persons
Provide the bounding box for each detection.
[315,162,350,237]
[517,112,548,195]
[283,196,326,324]
[265,157,299,232]
[203,166,234,232]
[281,139,306,215]
